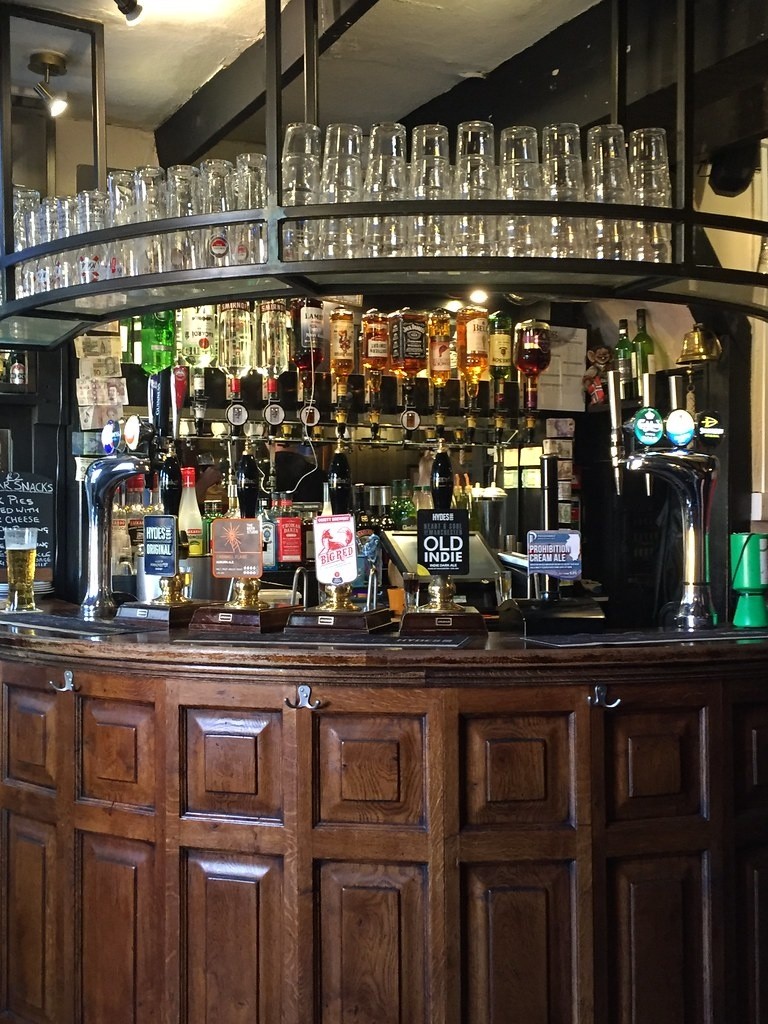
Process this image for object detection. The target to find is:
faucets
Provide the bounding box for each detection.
[605,370,721,634]
[79,416,183,619]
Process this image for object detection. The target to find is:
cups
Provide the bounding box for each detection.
[3,528,37,611]
[14,123,673,262]
[561,183,566,186]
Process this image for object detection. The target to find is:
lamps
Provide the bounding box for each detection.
[113,0,150,26]
[28,52,68,117]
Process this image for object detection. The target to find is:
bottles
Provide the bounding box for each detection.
[632,308,655,400]
[613,320,637,400]
[0,323,25,384]
[111,303,550,575]
